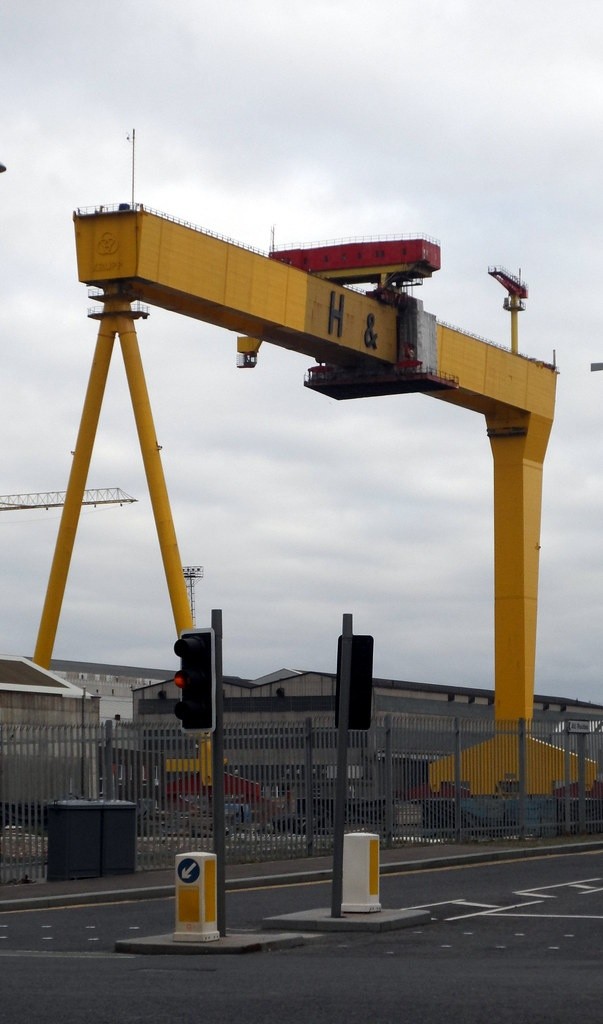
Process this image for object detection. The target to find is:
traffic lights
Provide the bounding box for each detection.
[332,635,374,731]
[173,627,220,731]
